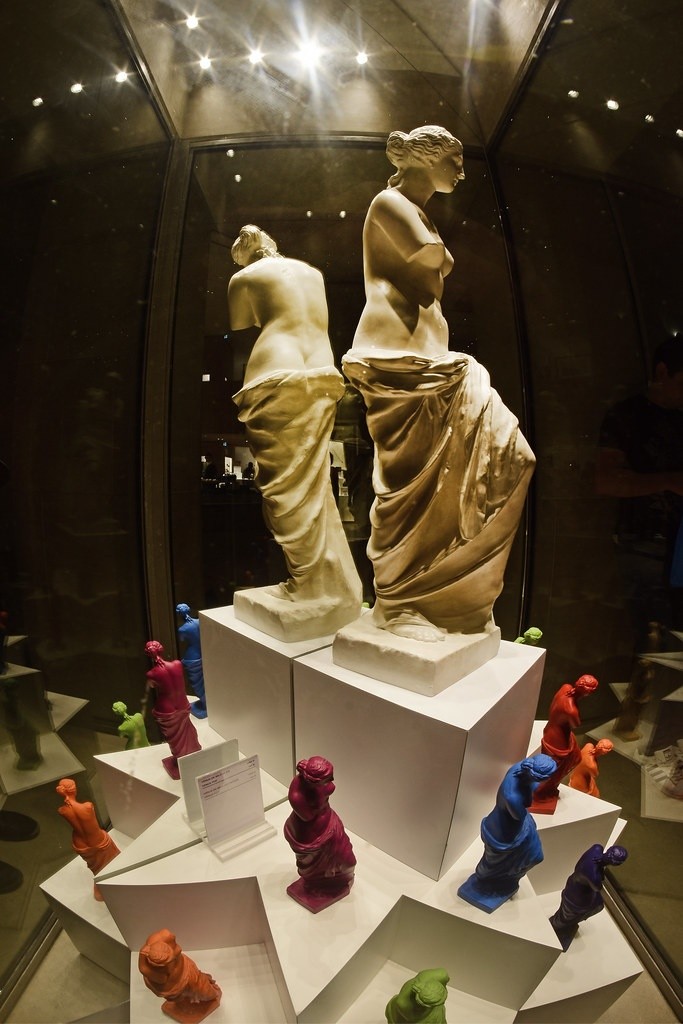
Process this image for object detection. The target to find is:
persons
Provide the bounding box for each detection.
[550,844,629,933]
[568,739,614,797]
[139,928,219,1004]
[57,779,120,875]
[386,968,450,1024]
[146,641,202,758]
[228,225,346,605]
[532,675,598,803]
[176,604,206,706]
[514,626,543,647]
[284,756,356,897]
[476,754,557,896]
[112,701,151,750]
[341,124,536,643]
